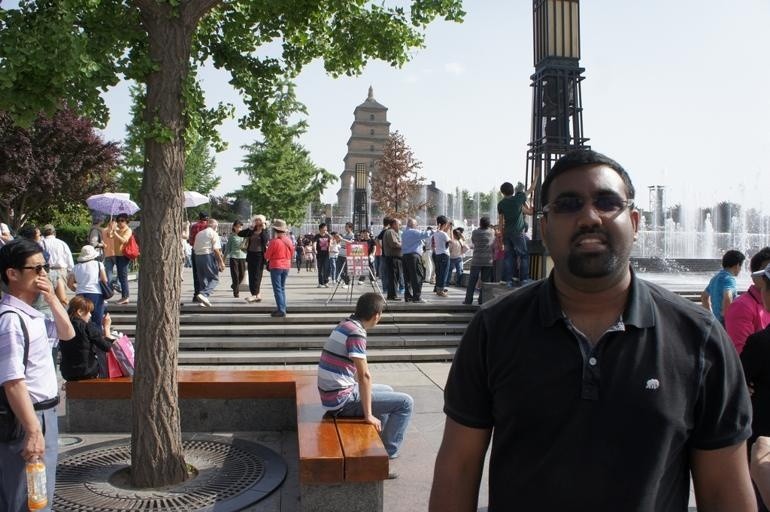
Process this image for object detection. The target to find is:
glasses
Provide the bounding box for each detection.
[24,264,50,274]
[543,193,634,213]
[117,219,125,223]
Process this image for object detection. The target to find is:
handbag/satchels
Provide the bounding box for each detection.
[110,334,135,376]
[107,351,122,378]
[0,388,25,443]
[122,235,139,260]
[99,281,114,299]
[94,245,104,261]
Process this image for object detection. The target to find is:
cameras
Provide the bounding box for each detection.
[353,233,363,242]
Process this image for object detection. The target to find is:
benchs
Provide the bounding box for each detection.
[65,365,388,512]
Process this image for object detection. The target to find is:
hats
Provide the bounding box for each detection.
[77,245,99,262]
[43,224,55,234]
[272,219,287,232]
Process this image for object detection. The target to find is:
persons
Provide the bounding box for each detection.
[0,213,133,380]
[489,166,541,288]
[425,148,760,512]
[462,216,497,304]
[0,238,77,511]
[182,212,465,318]
[316,291,414,481]
[701,247,770,511]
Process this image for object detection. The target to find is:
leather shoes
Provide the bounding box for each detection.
[116,298,128,305]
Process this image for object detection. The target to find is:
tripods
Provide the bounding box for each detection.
[324,258,387,304]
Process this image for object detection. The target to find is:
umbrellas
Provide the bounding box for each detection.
[85,193,141,221]
[184,190,210,208]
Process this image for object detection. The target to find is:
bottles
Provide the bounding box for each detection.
[24,455,48,511]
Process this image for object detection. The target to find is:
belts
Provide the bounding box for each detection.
[32,393,60,411]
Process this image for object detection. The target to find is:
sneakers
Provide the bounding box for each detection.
[272,311,284,317]
[197,294,211,307]
[318,280,447,303]
